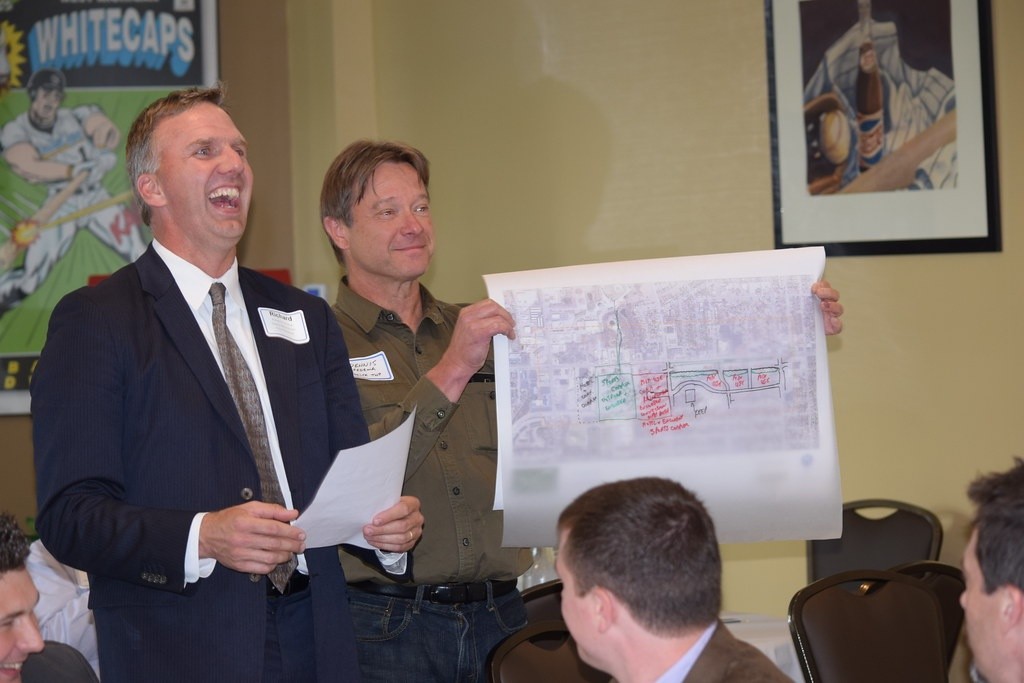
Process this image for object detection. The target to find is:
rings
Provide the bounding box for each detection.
[410,531,412,539]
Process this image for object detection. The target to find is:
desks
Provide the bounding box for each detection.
[719,611,807,683]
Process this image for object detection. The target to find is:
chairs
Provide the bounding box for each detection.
[485,497,968,683]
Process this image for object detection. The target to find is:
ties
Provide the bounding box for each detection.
[208,281,298,594]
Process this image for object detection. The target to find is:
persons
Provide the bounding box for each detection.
[948,458,1024,683]
[318,137,848,683]
[27,79,424,683]
[0,511,99,683]
[556,477,795,683]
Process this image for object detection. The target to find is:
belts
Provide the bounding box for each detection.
[263,572,311,599]
[355,578,519,603]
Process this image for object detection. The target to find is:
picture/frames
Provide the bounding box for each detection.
[766,0,1003,259]
